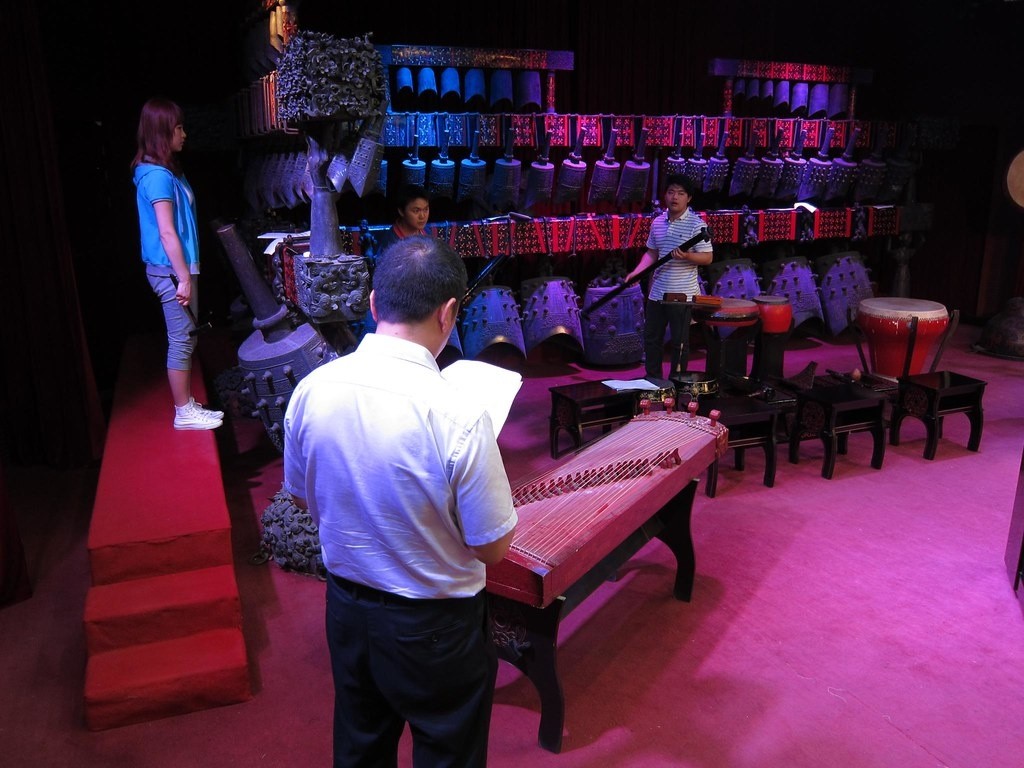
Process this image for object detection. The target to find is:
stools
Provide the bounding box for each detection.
[888,370,984,460]
[676,384,781,499]
[548,378,638,459]
[780,372,887,479]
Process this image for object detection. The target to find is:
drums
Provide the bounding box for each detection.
[752,294,794,333]
[692,296,761,326]
[669,369,719,396]
[1002,150,1024,213]
[858,297,950,394]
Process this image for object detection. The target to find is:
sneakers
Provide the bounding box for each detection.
[174,397,224,430]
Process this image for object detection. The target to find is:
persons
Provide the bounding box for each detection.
[283,235,519,768]
[625,175,713,379]
[1005,149,1024,611]
[131,95,225,431]
[366,184,472,329]
